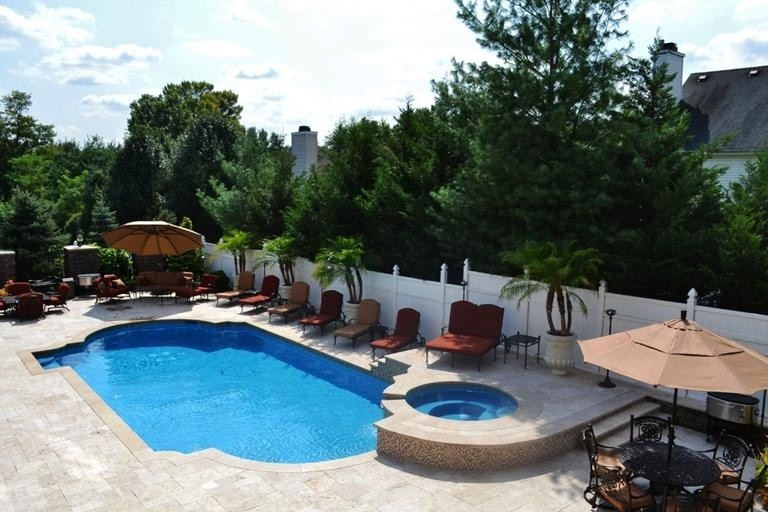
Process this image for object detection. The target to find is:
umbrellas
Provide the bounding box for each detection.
[98,220,205,303]
[578,309,768,467]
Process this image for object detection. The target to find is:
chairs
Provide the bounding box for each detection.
[134,272,193,304]
[12,292,45,322]
[582,421,621,486]
[333,298,381,350]
[238,275,281,317]
[451,304,505,370]
[104,273,132,301]
[370,308,422,361]
[425,300,477,367]
[44,282,70,313]
[700,429,751,491]
[658,483,720,512]
[7,282,32,296]
[266,282,309,324]
[175,274,216,304]
[628,411,669,444]
[703,465,767,511]
[215,271,255,307]
[298,290,343,336]
[92,277,117,304]
[590,457,654,512]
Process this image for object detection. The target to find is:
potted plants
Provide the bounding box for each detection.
[253,232,300,300]
[311,235,366,321]
[209,230,252,289]
[495,240,603,373]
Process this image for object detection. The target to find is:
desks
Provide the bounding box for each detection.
[503,331,541,370]
[0,292,49,318]
[618,441,720,511]
